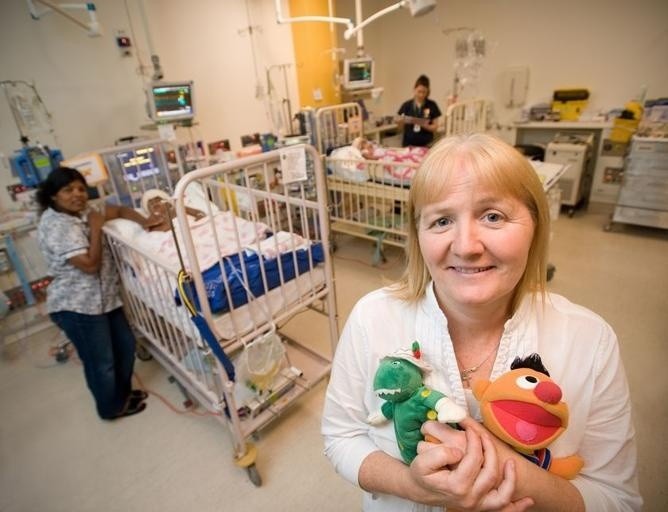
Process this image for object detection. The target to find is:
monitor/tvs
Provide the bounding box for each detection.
[115,144,165,196]
[147,79,194,123]
[344,58,375,91]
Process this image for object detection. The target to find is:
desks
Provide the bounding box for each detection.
[507,119,620,208]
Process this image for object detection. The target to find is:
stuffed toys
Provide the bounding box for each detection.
[363,338,468,471]
[472,350,586,482]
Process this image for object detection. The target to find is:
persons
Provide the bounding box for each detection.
[390,73,442,214]
[348,134,389,166]
[34,166,165,421]
[140,188,208,233]
[319,132,643,512]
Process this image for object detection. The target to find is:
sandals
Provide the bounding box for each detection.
[100,390,147,420]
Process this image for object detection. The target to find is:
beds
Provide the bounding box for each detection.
[314,100,491,254]
[86,148,342,457]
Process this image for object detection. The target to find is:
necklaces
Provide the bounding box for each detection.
[459,339,499,383]
